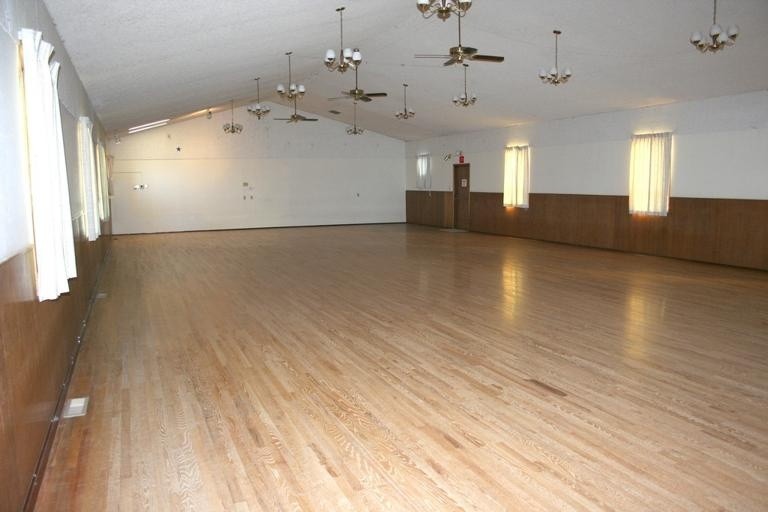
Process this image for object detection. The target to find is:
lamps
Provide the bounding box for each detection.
[538,29,571,85]
[324,7,359,73]
[223,98,243,135]
[247,76,271,120]
[394,83,416,119]
[452,63,477,106]
[416,1,472,22]
[345,103,365,135]
[277,51,306,103]
[688,1,739,53]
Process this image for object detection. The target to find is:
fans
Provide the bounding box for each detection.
[273,96,318,123]
[414,11,505,66]
[327,68,387,102]
